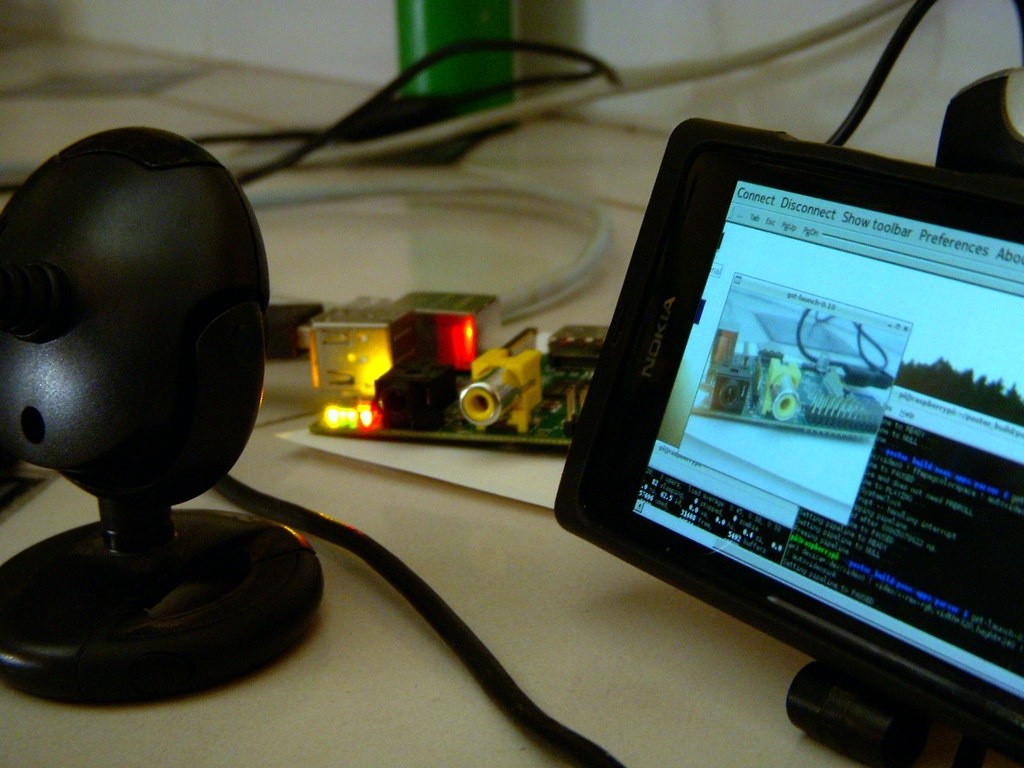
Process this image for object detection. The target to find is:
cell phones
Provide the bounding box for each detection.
[551,118,1024,754]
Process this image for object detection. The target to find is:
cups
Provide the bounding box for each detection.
[395,0,514,115]
